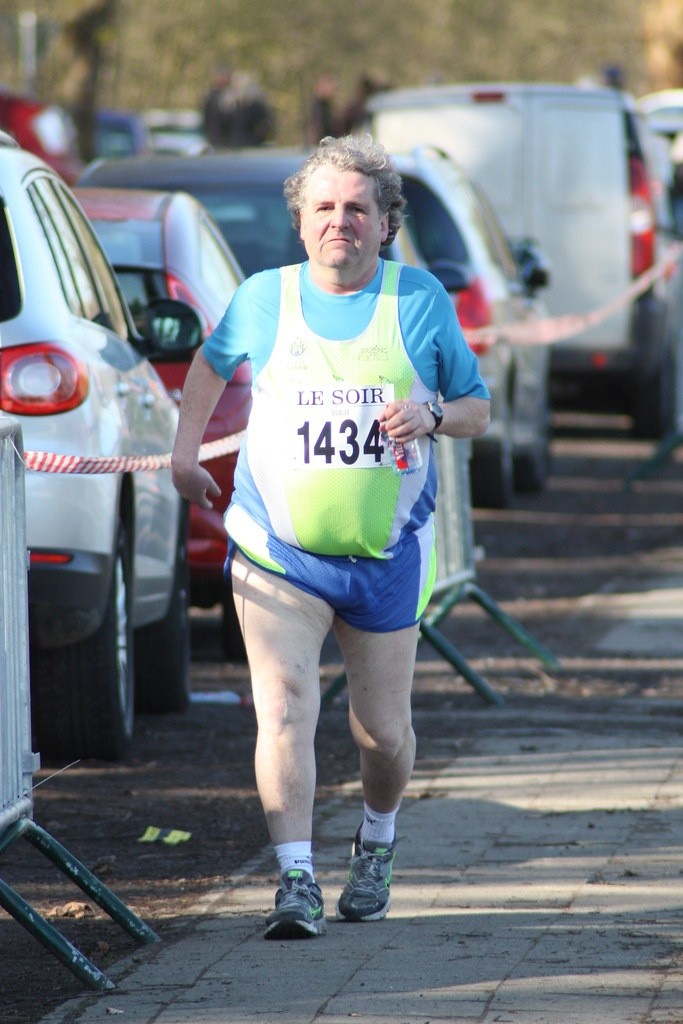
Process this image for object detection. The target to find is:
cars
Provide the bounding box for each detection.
[63,183,252,666]
[0,126,191,766]
[0,81,683,505]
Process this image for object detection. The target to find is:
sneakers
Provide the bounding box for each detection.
[263,869,327,938]
[336,821,397,922]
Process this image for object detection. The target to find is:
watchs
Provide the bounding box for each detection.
[421,401,443,443]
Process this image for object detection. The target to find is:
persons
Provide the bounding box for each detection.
[199,72,380,151]
[171,137,490,940]
